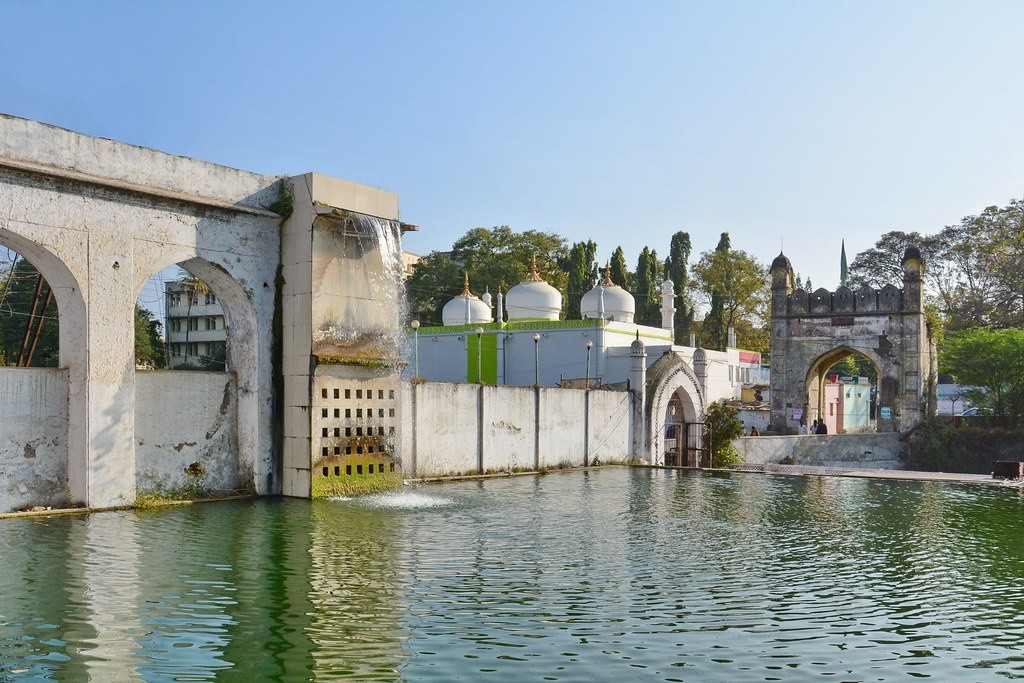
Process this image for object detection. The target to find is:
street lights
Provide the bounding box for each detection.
[476,326,484,383]
[585,340,593,390]
[411,320,421,378]
[532,333,540,388]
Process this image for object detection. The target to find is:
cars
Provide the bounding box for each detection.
[952,406,996,417]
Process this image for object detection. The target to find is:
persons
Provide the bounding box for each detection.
[811,421,819,435]
[798,419,809,436]
[751,427,761,437]
[817,419,828,435]
